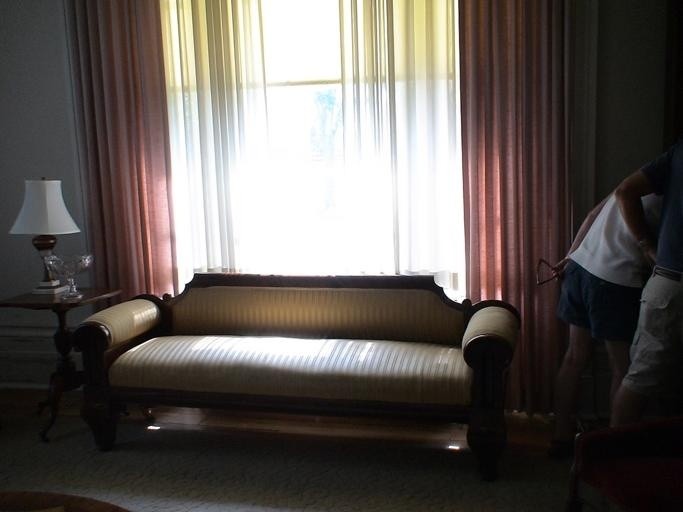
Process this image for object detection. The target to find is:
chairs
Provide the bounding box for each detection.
[565,415,683,511]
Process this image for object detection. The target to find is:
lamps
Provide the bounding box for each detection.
[8,177,81,295]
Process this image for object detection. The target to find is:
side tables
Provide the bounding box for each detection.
[0,286,130,443]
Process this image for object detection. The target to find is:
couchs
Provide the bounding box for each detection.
[72,273,522,471]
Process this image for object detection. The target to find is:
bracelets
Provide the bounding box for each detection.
[638,232,657,247]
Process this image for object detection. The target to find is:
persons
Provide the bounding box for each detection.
[548,169,667,450]
[608,137,683,448]
